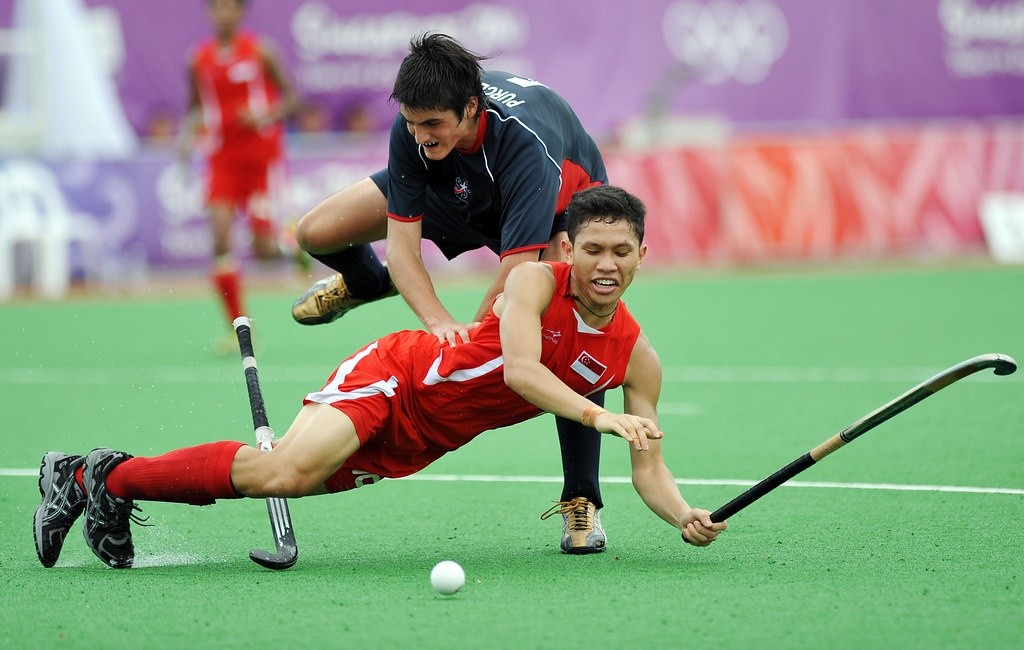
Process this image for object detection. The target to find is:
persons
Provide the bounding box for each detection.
[34,185,727,570]
[178,0,301,351]
[291,30,609,555]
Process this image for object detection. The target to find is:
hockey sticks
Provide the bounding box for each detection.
[231,313,301,572]
[681,352,1020,551]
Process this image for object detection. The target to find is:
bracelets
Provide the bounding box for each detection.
[581,404,607,428]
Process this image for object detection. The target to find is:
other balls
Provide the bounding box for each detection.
[430,561,468,599]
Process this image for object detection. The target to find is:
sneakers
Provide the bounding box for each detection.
[540,498,607,552]
[80,448,155,569]
[293,261,401,325]
[33,452,88,567]
